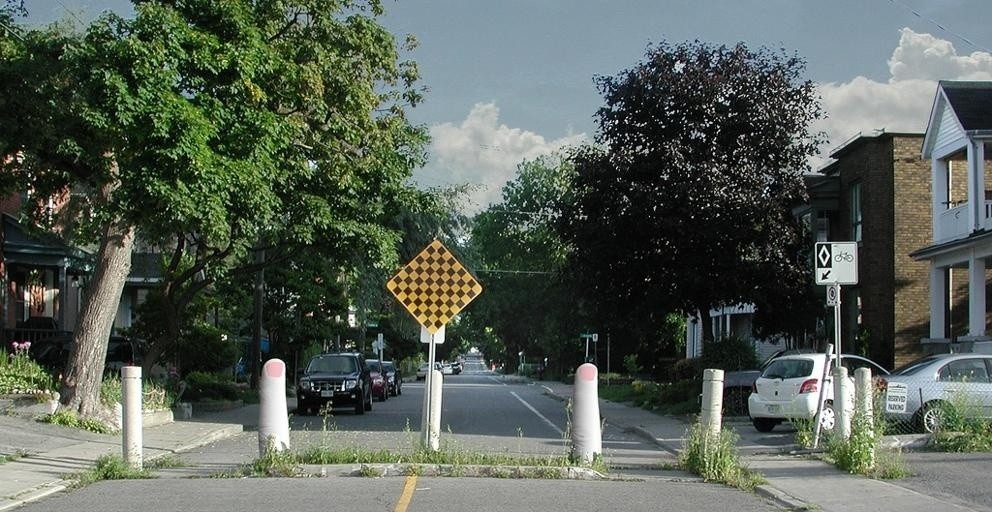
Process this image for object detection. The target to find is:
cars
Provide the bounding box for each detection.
[259,338,274,363]
[443,359,466,376]
[367,359,387,399]
[747,352,889,432]
[874,353,992,432]
[381,360,405,396]
[416,363,444,381]
[29,336,163,376]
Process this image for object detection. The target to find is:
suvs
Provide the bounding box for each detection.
[726,349,791,417]
[295,353,375,415]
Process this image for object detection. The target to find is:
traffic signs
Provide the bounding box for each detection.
[580,333,594,338]
